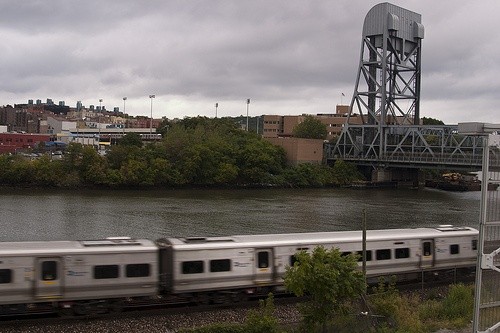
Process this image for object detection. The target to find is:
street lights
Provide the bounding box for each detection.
[123,96,127,137]
[149,95,156,134]
[216,103,218,118]
[99,98,103,137]
[246,97,251,132]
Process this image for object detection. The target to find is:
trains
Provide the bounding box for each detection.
[0,224,482,321]
